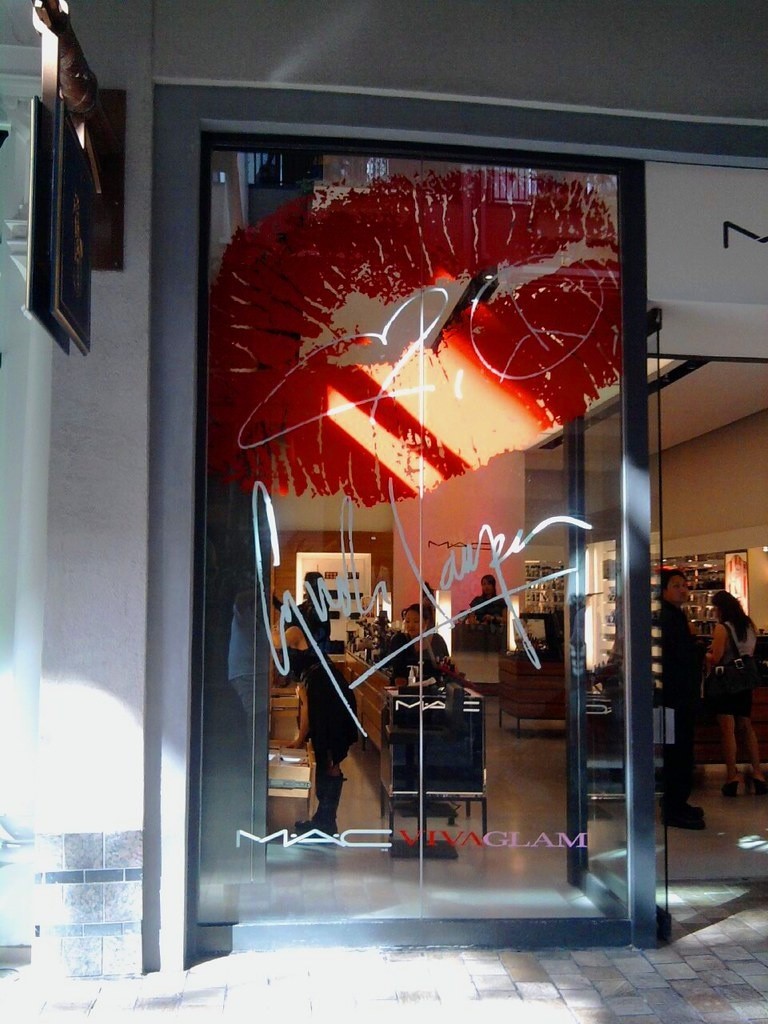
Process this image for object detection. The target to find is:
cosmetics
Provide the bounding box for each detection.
[395,654,467,691]
[525,562,564,614]
[324,571,401,666]
[678,565,725,645]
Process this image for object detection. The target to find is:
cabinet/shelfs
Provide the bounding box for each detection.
[268,530,748,838]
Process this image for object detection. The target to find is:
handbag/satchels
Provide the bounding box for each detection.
[711,622,761,696]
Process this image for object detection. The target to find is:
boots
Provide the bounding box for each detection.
[295,769,347,845]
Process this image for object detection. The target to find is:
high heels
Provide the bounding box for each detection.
[721,772,745,796]
[750,775,768,794]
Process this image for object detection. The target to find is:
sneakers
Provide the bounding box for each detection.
[659,797,706,830]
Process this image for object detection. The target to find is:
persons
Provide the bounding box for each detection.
[705,589,768,797]
[662,568,705,830]
[271,604,359,846]
[389,604,450,686]
[272,570,331,649]
[468,574,508,624]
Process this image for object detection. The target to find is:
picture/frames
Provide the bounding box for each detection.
[23,96,70,356]
[53,99,93,355]
